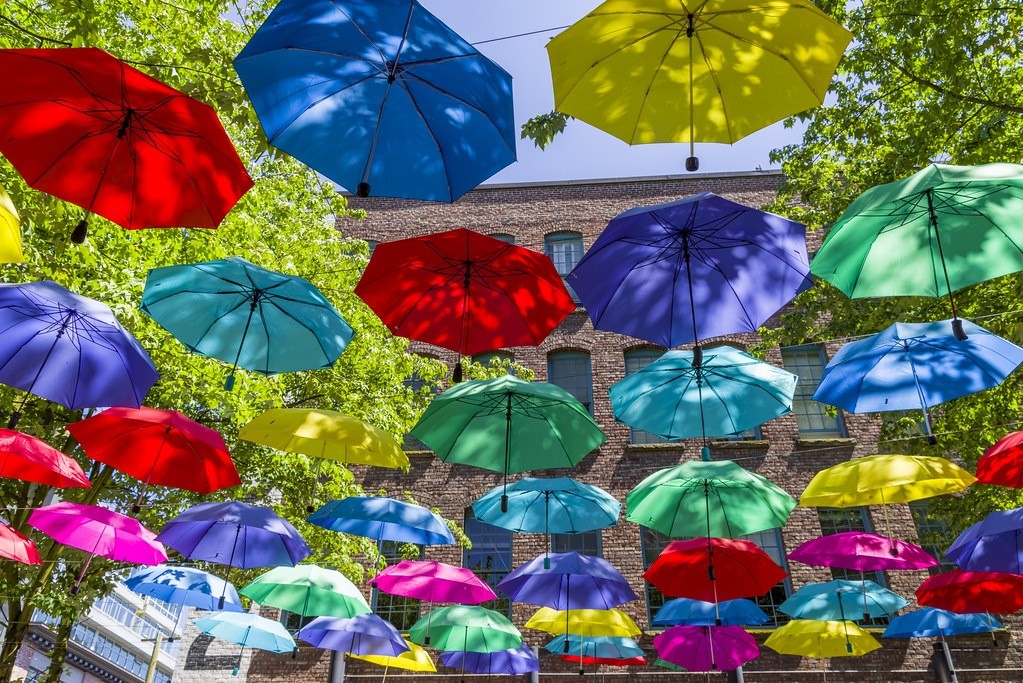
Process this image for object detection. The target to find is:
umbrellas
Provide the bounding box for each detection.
[0,0,1023,683]
[787,530,941,623]
